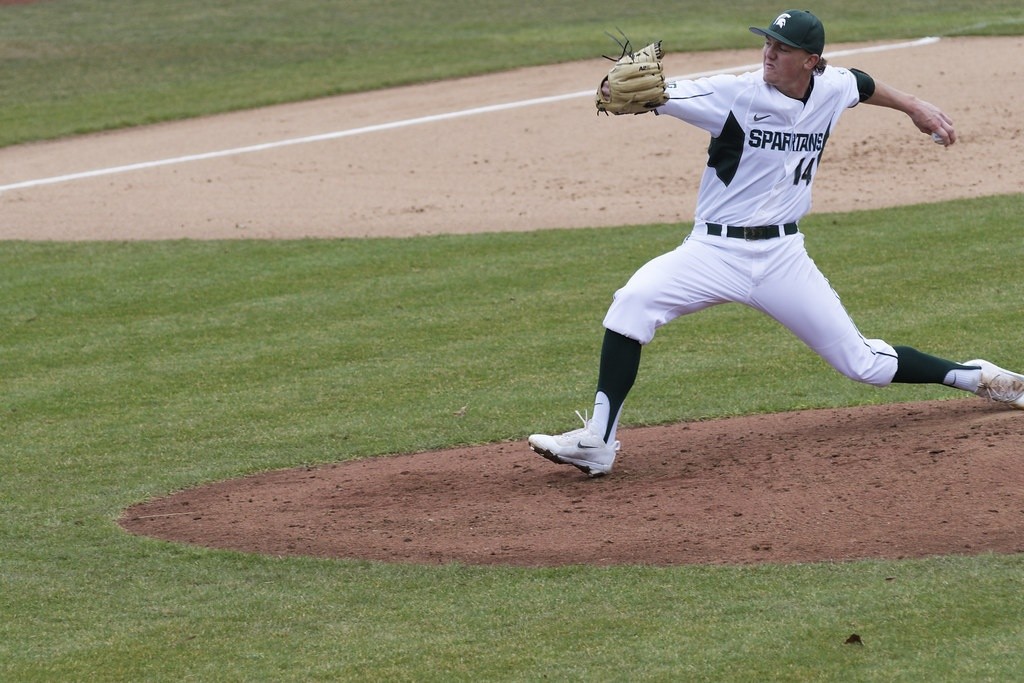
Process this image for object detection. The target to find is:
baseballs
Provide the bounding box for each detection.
[931,130,946,145]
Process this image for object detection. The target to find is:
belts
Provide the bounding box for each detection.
[706,221,798,239]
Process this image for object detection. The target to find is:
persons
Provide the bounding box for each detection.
[526,9,1024,477]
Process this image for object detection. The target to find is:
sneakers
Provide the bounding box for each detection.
[968,359,1023,409]
[526,421,620,478]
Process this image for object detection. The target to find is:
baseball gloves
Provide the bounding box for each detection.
[595,25,669,123]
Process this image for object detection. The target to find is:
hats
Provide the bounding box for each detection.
[748,9,825,58]
